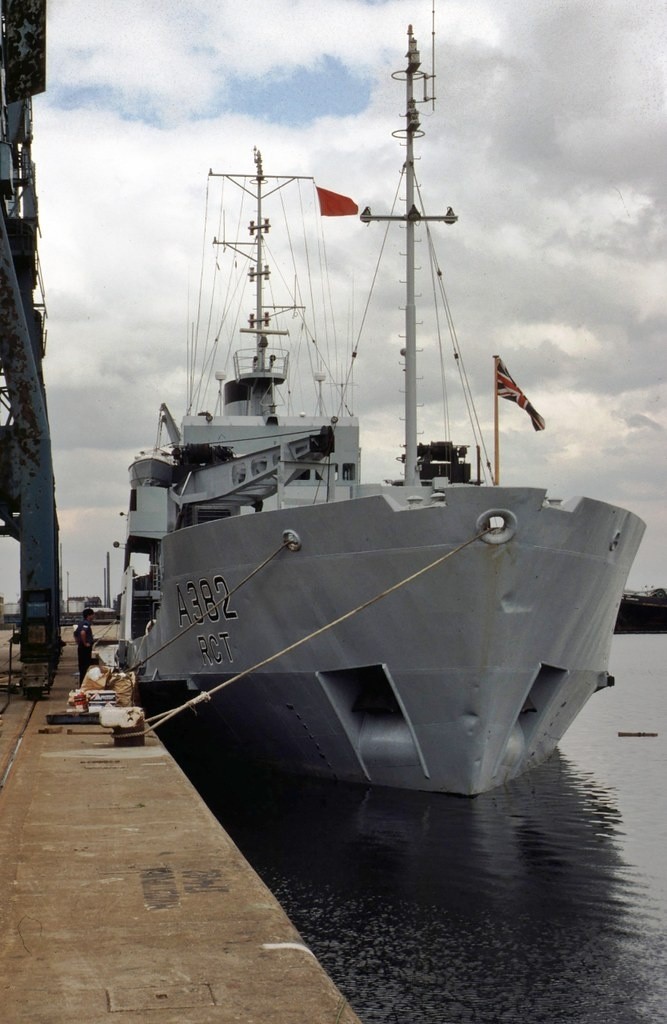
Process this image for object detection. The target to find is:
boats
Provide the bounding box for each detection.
[111,0,650,798]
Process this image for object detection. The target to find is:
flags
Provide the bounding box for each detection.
[497,357,545,432]
[316,186,358,216]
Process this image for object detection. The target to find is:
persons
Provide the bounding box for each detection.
[73,609,94,688]
